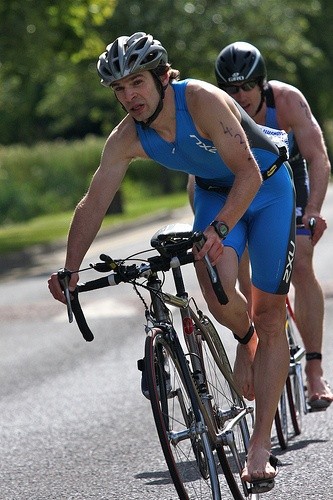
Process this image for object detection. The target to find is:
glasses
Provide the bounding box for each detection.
[223,81,256,95]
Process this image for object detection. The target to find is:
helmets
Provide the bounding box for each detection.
[96,32,169,88]
[214,42,266,86]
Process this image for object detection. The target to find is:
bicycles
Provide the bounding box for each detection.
[275,213,318,450]
[56,223,256,500]
